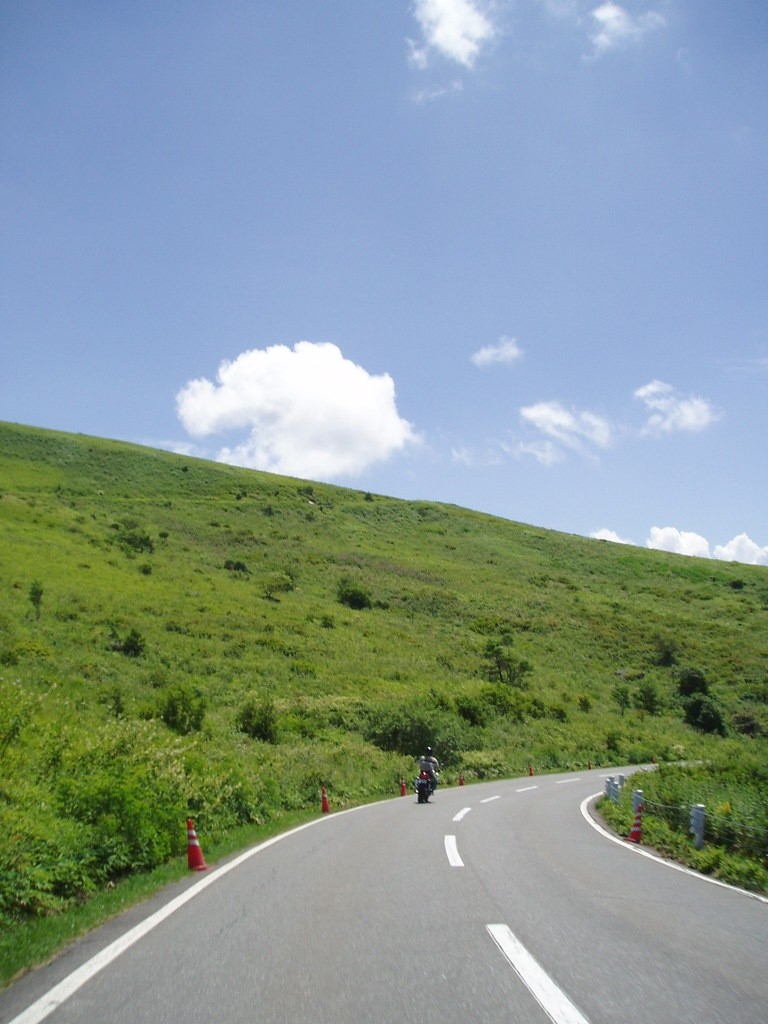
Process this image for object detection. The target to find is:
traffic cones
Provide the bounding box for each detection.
[623,804,643,843]
[653,757,656,764]
[459,771,465,786]
[322,786,331,813]
[529,766,534,776]
[400,776,407,797]
[186,817,208,870]
[588,760,591,769]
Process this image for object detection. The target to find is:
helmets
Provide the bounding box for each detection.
[424,747,432,756]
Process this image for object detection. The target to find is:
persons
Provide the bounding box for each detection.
[415,746,440,795]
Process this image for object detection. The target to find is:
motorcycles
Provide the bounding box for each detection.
[414,763,447,804]
